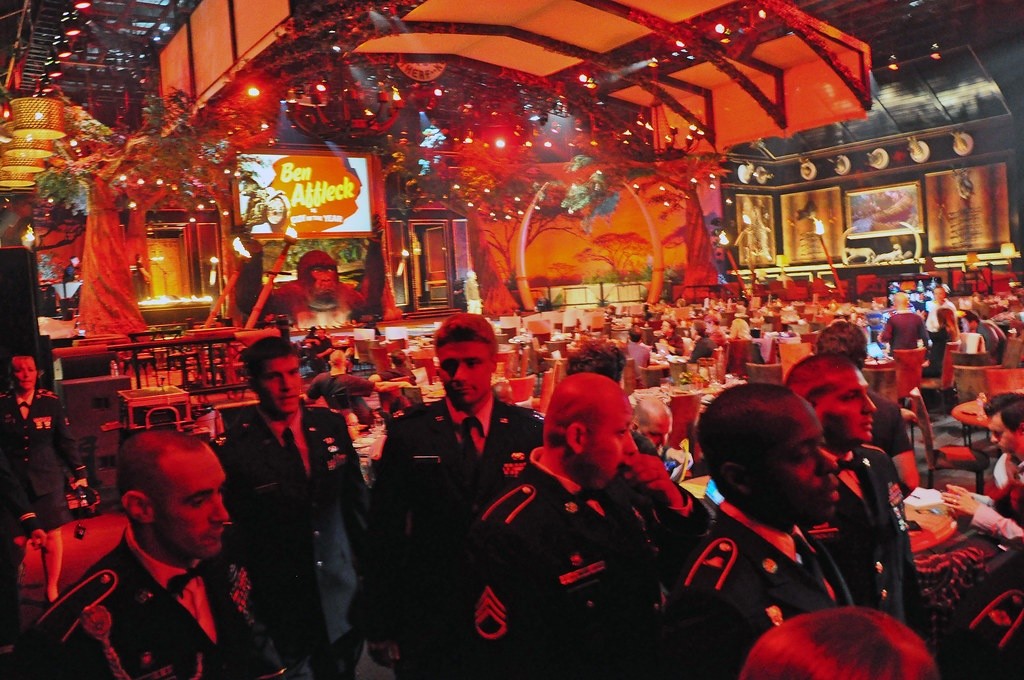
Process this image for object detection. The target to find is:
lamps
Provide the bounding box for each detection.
[0,98,65,187]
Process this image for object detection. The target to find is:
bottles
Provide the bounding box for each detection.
[109,361,119,378]
[18,538,44,626]
[697,345,725,383]
[494,363,506,396]
[977,392,986,416]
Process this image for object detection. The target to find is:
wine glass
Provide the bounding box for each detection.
[374,418,386,438]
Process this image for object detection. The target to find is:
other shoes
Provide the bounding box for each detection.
[19,562,26,589]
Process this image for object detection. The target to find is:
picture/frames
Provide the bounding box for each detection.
[845,179,925,240]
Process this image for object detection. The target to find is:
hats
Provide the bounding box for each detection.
[331,351,347,376]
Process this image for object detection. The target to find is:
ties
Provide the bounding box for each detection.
[791,534,834,604]
[281,426,307,478]
[461,418,485,462]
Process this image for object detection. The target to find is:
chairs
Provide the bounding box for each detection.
[52,285,1024,501]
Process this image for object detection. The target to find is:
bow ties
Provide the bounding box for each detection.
[836,459,858,471]
[575,490,600,502]
[166,560,210,601]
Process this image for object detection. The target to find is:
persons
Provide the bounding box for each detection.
[0,286,1024,680]
[6,430,282,679]
[358,310,544,679]
[208,336,380,680]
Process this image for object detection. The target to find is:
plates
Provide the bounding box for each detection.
[353,437,373,448]
